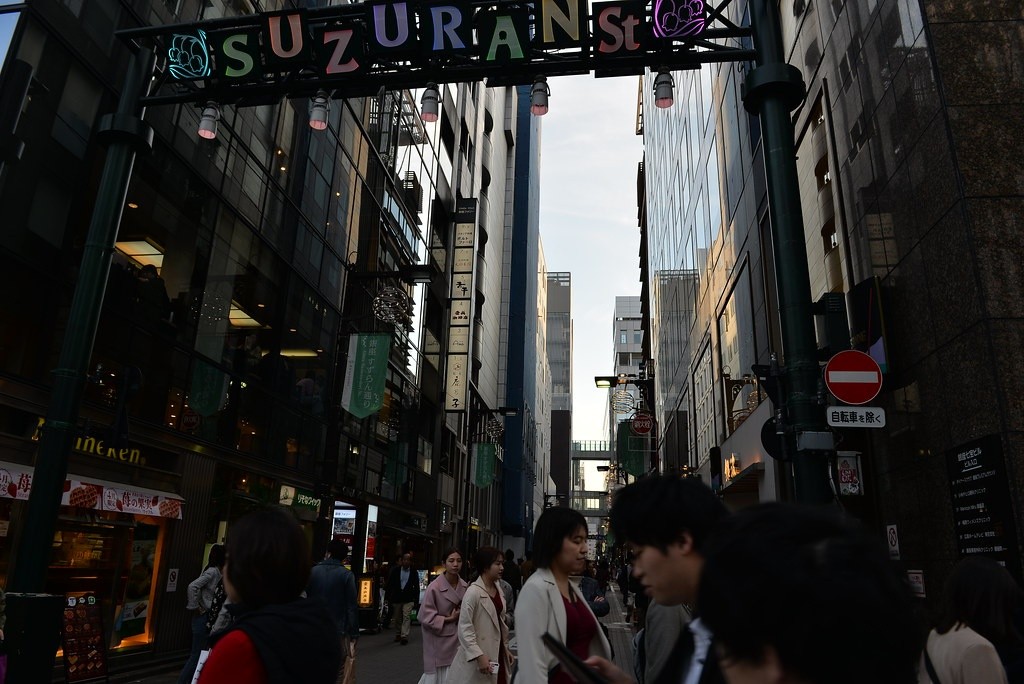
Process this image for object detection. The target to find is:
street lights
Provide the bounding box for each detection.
[594,153,659,479]
[462,406,521,565]
[597,466,628,487]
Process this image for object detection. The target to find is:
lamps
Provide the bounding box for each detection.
[528,79,553,116]
[419,83,442,122]
[652,73,676,109]
[308,93,334,130]
[197,101,219,139]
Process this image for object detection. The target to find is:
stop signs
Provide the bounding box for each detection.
[825,349,884,404]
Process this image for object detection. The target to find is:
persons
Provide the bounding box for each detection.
[513,506,612,684]
[563,477,1024,684]
[177,505,359,684]
[420,545,522,684]
[374,554,420,645]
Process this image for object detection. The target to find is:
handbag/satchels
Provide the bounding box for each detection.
[342,642,355,684]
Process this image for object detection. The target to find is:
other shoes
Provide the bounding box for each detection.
[393,635,401,643]
[401,639,408,645]
[382,622,391,630]
